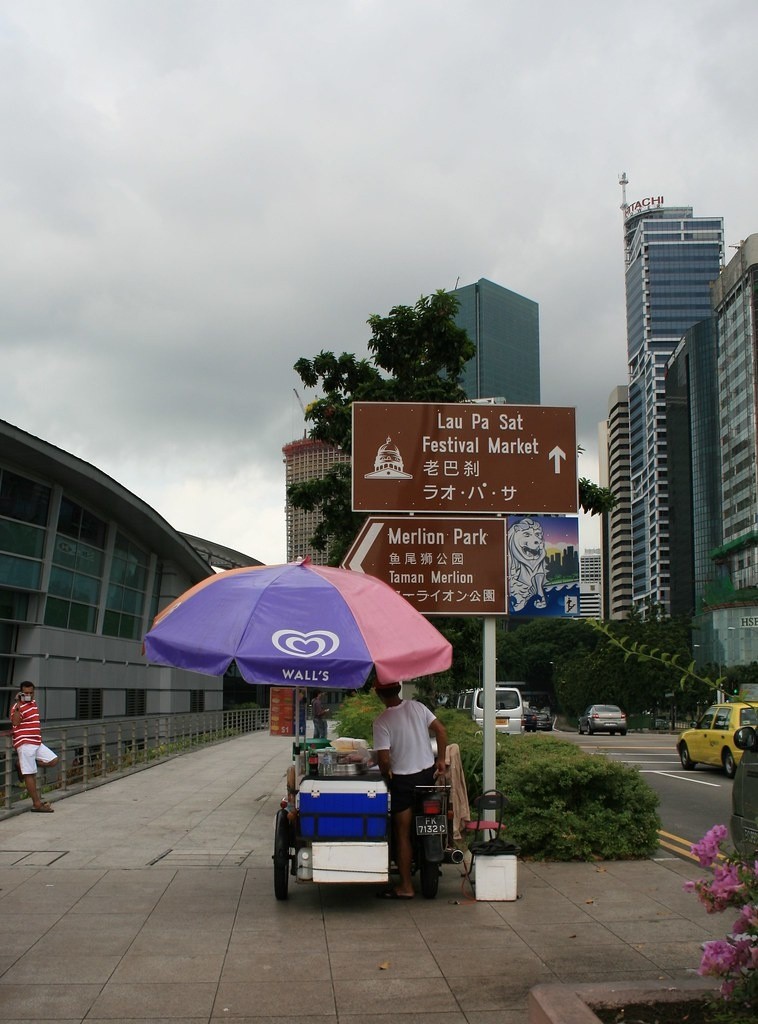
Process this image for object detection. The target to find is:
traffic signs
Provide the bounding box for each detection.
[339,402,582,619]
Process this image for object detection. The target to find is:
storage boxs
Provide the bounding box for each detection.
[312,841,389,882]
[299,779,388,841]
[475,855,517,901]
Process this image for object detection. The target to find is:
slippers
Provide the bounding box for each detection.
[375,888,414,899]
[31,802,54,812]
[14,761,24,783]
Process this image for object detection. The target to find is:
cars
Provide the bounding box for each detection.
[675,700,758,780]
[521,707,553,733]
[577,705,628,736]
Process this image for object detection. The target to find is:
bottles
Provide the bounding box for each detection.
[296,847,313,880]
[323,749,333,776]
[308,743,319,776]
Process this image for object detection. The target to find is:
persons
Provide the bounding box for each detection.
[373,678,448,898]
[10,681,59,813]
[311,690,330,739]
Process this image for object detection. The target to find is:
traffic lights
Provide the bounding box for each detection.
[732,680,740,696]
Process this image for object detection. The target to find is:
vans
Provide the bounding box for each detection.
[730,724,758,859]
[445,686,524,736]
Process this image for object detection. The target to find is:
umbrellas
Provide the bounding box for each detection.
[144,556,453,792]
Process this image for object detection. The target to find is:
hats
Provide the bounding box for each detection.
[374,676,399,689]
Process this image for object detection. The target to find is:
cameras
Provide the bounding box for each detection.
[19,693,32,702]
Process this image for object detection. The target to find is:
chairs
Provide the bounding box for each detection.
[459,788,509,883]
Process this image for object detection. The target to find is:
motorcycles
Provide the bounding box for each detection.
[272,775,463,900]
